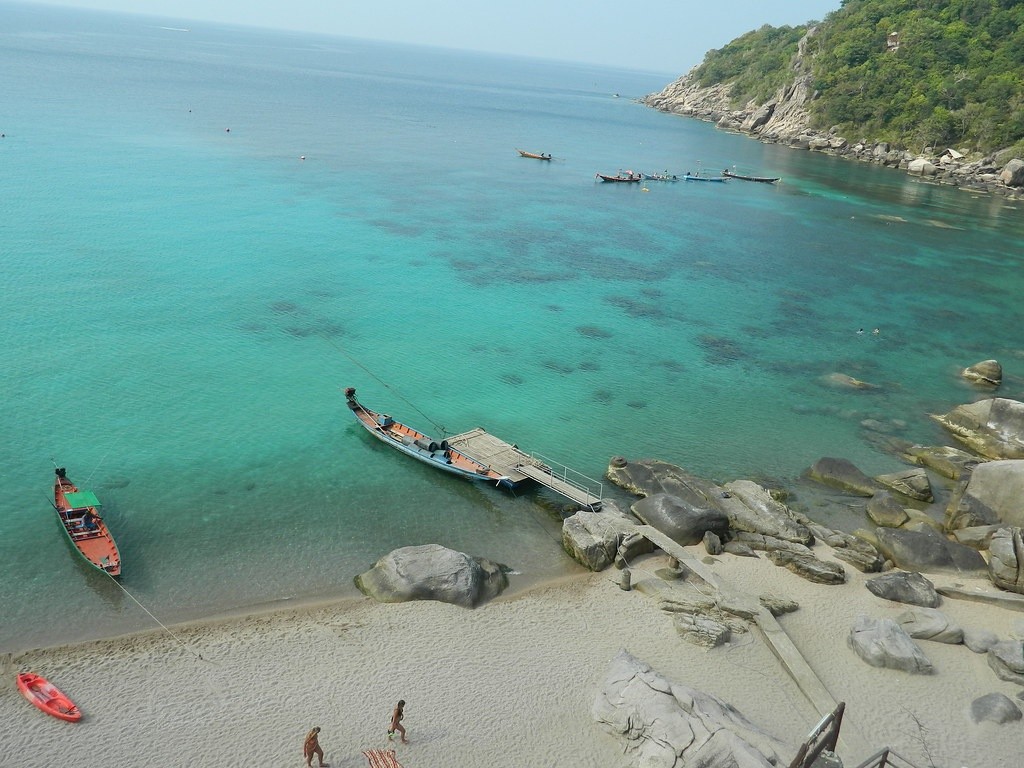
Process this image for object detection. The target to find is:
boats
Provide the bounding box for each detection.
[515,146,552,160]
[345,386,514,492]
[723,169,780,182]
[643,173,680,181]
[53,466,122,576]
[16,670,83,723]
[684,173,729,181]
[596,173,643,183]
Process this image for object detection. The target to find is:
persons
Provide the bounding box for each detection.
[388,700,408,744]
[541,153,545,158]
[303,726,329,768]
[617,168,699,180]
[82,511,103,531]
